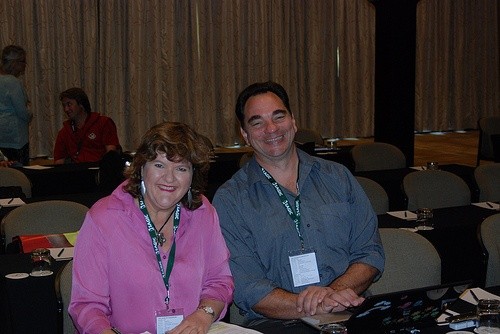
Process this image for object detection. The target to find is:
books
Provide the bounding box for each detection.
[47,247,74,261]
[0,198,25,207]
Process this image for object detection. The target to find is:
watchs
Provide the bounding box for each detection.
[198,306,214,317]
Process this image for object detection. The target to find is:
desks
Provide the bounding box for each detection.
[0,145,356,208]
[0,249,80,334]
[246,280,500,334]
[350,163,480,211]
[376,202,500,291]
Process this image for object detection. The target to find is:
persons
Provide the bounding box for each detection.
[212,81,385,327]
[0,45,33,169]
[54,88,120,162]
[67,122,236,334]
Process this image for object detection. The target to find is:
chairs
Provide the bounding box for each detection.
[337,115,500,297]
[0,168,89,334]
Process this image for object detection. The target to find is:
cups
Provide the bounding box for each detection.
[425,161,440,170]
[417,207,433,231]
[320,323,347,334]
[327,142,337,149]
[30,248,51,276]
[477,300,500,334]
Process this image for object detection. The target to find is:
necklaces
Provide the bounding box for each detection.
[147,206,176,247]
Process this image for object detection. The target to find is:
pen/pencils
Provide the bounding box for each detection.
[58,249,64,257]
[470,290,479,303]
[8,199,13,204]
[112,327,120,334]
[405,212,407,217]
[487,203,493,208]
